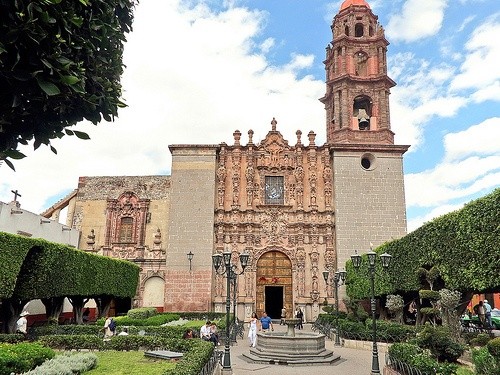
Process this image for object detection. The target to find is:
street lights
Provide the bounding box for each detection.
[322,270,346,347]
[351,248,393,375]
[211,249,250,370]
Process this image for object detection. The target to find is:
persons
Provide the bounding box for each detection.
[257,312,274,333]
[82,307,90,322]
[182,329,193,339]
[294,308,303,330]
[99,311,117,342]
[247,312,261,348]
[16,310,30,336]
[199,321,221,349]
[473,300,492,329]
[279,306,287,325]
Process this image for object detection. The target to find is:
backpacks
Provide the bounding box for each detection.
[107,319,117,331]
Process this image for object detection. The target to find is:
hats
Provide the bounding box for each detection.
[483,300,488,302]
[20,311,29,316]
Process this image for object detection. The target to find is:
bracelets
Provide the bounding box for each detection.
[272,328,273,329]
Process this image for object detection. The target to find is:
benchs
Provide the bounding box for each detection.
[311,321,332,339]
[461,320,494,334]
[219,317,245,345]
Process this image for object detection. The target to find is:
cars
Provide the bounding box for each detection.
[471,308,500,331]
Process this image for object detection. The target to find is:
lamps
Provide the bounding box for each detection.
[187,252,194,269]
